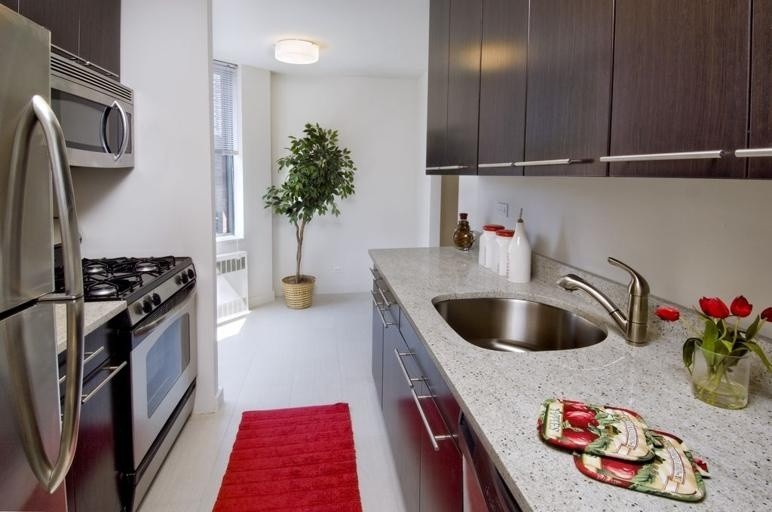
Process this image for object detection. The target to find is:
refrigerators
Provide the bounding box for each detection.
[0,0,84,512]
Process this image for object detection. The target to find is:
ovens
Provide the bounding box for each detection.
[128,263,198,512]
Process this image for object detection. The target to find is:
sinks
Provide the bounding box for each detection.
[436,299,607,352]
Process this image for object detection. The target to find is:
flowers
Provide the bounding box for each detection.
[654,294,772,355]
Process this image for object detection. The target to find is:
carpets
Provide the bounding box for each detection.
[211,403,363,512]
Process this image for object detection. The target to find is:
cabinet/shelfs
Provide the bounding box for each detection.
[476,0,616,175]
[370,263,401,410]
[609,0,772,182]
[1,0,122,81]
[425,0,483,175]
[379,298,525,512]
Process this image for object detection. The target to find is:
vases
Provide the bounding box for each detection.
[692,341,755,410]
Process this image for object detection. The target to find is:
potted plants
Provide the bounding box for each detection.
[262,123,357,309]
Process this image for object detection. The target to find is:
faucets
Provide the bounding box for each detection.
[555,257,651,347]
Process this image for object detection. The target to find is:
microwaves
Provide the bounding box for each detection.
[51,51,136,168]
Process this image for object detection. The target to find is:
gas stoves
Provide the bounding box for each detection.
[57,255,196,305]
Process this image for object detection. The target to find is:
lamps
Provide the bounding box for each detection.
[274,39,321,65]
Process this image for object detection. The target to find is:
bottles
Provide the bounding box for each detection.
[477,225,506,268]
[494,229,514,277]
[507,218,531,284]
